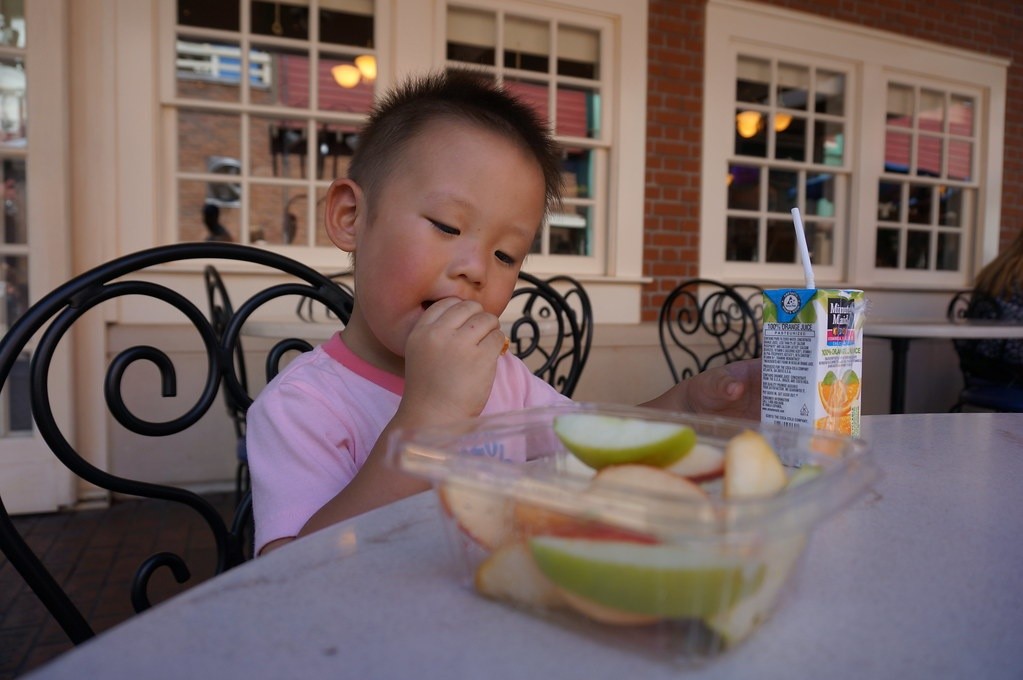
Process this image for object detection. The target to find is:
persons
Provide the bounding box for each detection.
[1,2,31,407]
[955,219,1023,414]
[199,201,298,245]
[244,71,770,560]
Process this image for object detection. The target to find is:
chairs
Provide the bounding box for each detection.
[656,278,771,388]
[0,233,598,649]
[947,287,1023,411]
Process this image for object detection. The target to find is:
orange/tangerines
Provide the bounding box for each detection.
[814,378,860,456]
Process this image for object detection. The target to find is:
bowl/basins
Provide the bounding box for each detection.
[397,398,883,667]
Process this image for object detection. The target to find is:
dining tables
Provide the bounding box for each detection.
[862,314,1023,412]
[23,402,1023,680]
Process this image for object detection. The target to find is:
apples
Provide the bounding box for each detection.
[438,415,822,645]
